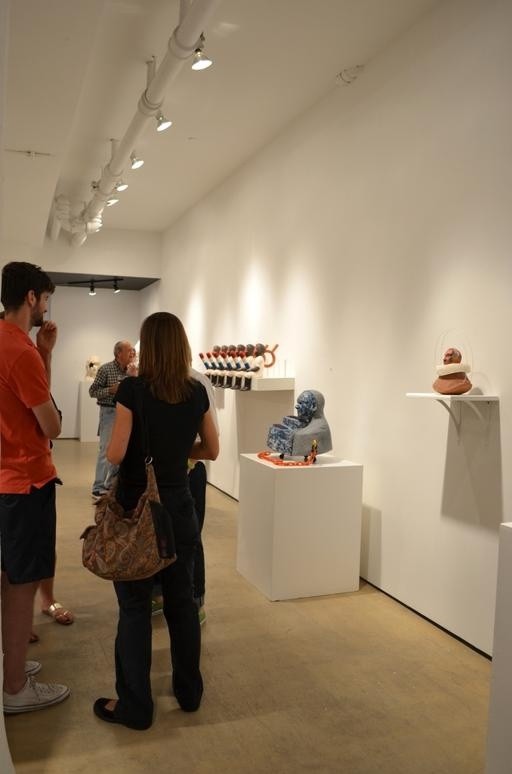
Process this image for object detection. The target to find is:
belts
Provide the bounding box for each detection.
[100,403,116,408]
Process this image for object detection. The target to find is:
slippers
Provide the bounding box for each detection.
[42,602,73,624]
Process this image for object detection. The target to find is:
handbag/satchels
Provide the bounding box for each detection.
[81,493,176,582]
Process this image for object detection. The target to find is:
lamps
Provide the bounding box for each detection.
[87,280,121,295]
[114,46,214,191]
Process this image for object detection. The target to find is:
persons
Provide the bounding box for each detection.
[265,387,333,458]
[86,337,140,498]
[28,394,77,647]
[430,347,472,396]
[92,310,222,732]
[0,258,72,717]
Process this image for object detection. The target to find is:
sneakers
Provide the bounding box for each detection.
[93,697,152,729]
[93,488,109,499]
[4,676,70,713]
[150,600,206,628]
[24,661,42,674]
